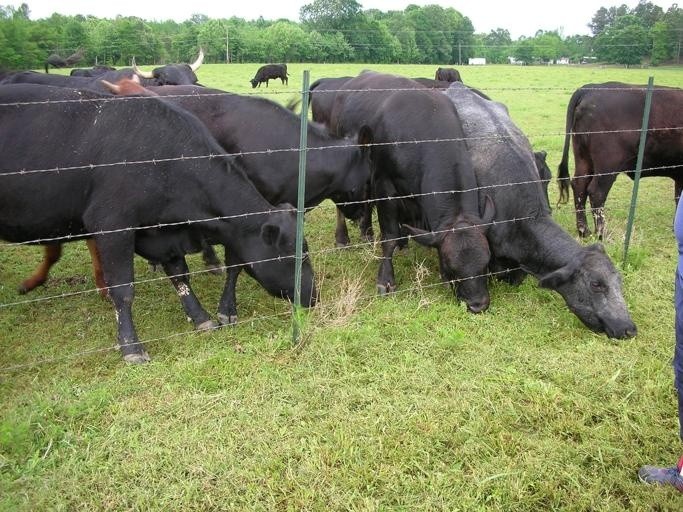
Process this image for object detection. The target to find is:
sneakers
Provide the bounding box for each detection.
[637,465,683,495]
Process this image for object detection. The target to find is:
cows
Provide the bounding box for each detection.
[556,79,683,241]
[2,82,321,363]
[146,84,384,276]
[3,44,208,97]
[409,76,639,342]
[409,67,552,218]
[249,64,290,89]
[303,74,379,247]
[328,69,497,317]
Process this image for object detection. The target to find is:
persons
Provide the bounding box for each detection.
[637,190,683,497]
